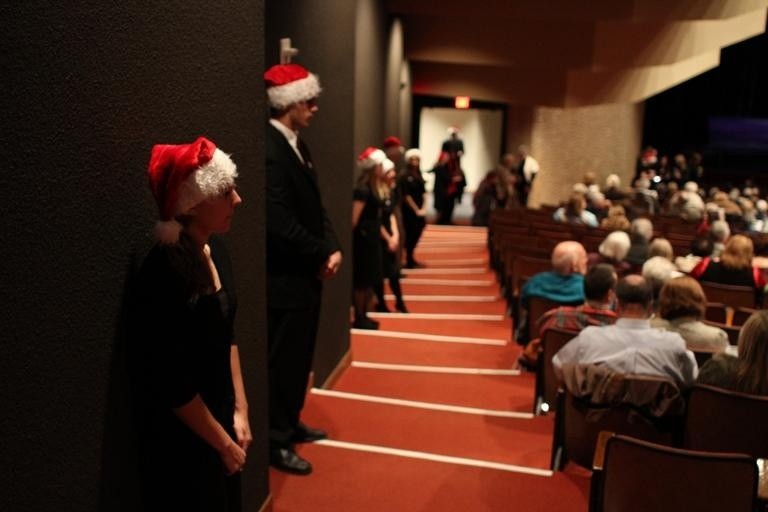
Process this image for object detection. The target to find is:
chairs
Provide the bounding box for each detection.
[486,201,768,512]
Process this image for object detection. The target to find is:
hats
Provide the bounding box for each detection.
[147,137,240,249]
[355,136,422,178]
[264,62,323,110]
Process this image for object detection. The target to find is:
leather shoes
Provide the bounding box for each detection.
[270,448,312,474]
[355,300,407,330]
[407,261,427,269]
[301,427,326,443]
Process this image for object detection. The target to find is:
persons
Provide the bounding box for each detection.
[108,135,253,511]
[511,145,768,401]
[353,136,429,330]
[263,64,344,476]
[433,132,467,225]
[471,141,538,228]
